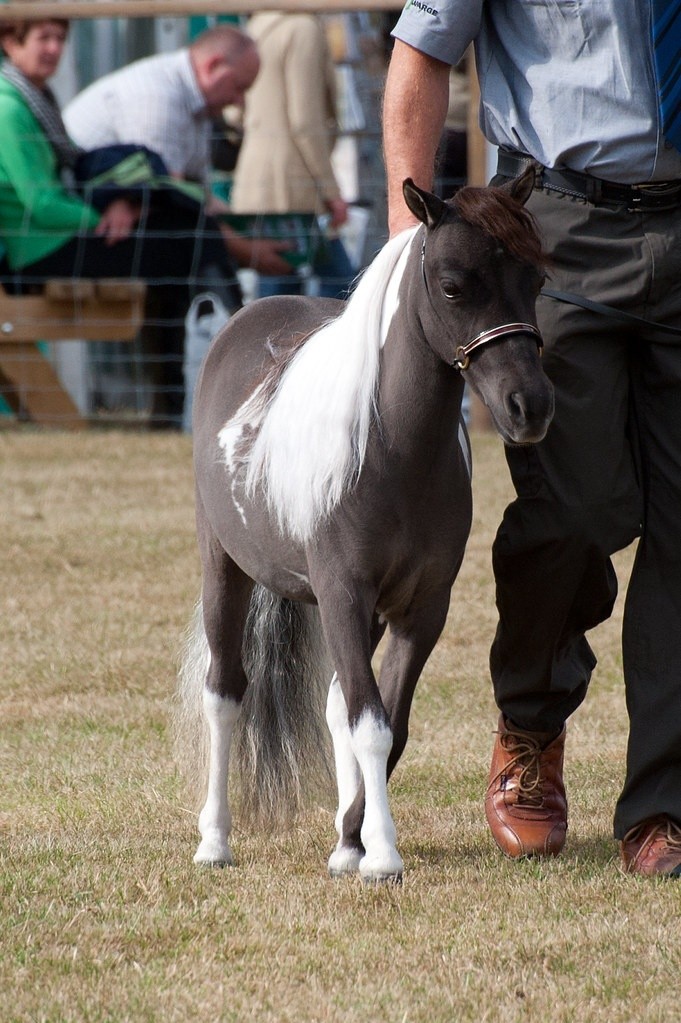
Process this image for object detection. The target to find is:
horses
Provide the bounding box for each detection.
[174,160,556,883]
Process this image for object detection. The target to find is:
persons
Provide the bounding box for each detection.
[59,24,295,279]
[1,0,242,430]
[379,0,680,878]
[220,11,359,301]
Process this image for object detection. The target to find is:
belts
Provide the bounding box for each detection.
[492,144,680,221]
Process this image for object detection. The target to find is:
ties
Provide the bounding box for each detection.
[646,0,681,153]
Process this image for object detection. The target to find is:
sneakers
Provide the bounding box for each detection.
[614,812,679,882]
[482,704,571,864]
